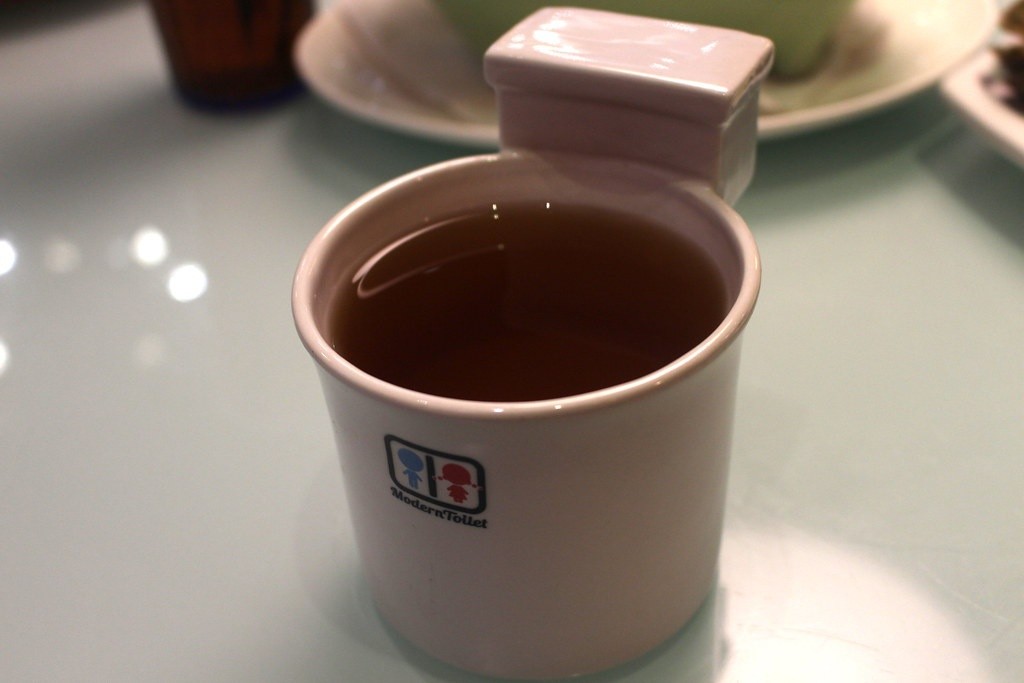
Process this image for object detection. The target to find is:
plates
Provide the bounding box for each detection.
[942,0,1024,166]
[292,0,1002,147]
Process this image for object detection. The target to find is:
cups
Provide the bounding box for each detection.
[294,5,779,680]
[148,0,312,114]
